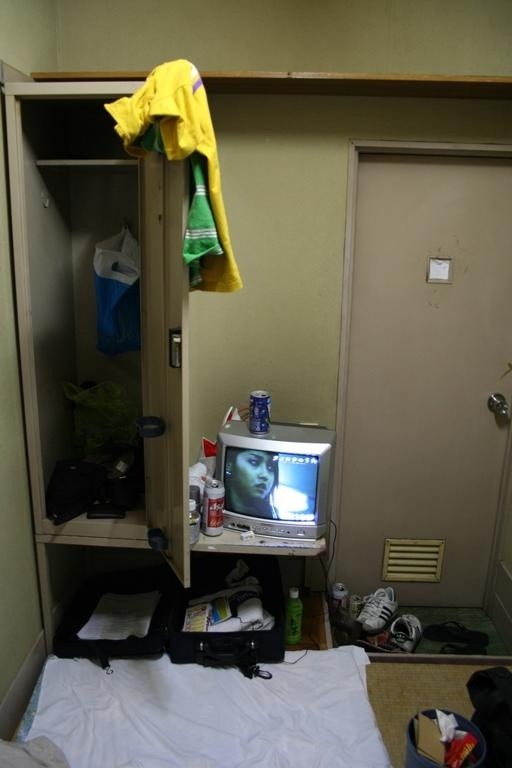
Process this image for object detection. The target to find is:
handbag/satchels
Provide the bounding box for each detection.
[91,226,142,358]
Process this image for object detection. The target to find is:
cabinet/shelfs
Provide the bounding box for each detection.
[2,79,162,545]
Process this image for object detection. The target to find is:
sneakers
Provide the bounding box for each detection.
[386,612,423,653]
[355,584,400,633]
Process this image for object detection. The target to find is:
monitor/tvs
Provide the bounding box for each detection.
[216,421,336,542]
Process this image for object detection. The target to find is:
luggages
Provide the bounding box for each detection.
[52,556,286,679]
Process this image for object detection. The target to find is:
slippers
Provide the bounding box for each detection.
[423,618,491,655]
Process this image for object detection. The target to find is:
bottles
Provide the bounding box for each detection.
[286,586,304,645]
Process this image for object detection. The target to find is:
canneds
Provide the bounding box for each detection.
[333,584,347,609]
[249,390,272,436]
[201,480,225,536]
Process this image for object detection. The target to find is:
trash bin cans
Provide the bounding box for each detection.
[406,709,487,767]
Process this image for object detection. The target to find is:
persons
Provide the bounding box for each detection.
[226,448,279,521]
